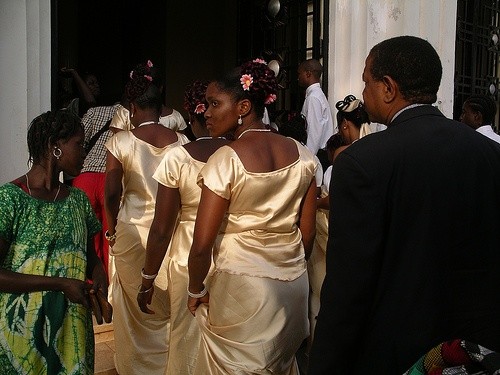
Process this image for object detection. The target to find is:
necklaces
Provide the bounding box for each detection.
[195,136,226,142]
[26,173,61,201]
[138,121,162,127]
[238,129,271,140]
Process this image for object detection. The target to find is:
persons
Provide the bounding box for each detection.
[0,109,108,375]
[62,36,500,375]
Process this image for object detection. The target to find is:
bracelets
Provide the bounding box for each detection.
[137,283,154,293]
[105,230,117,241]
[140,268,158,280]
[187,287,207,298]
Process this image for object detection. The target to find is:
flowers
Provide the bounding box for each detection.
[147,60,153,67]
[144,74,153,81]
[265,94,277,105]
[253,58,268,65]
[240,74,254,91]
[194,104,206,114]
[130,70,133,79]
